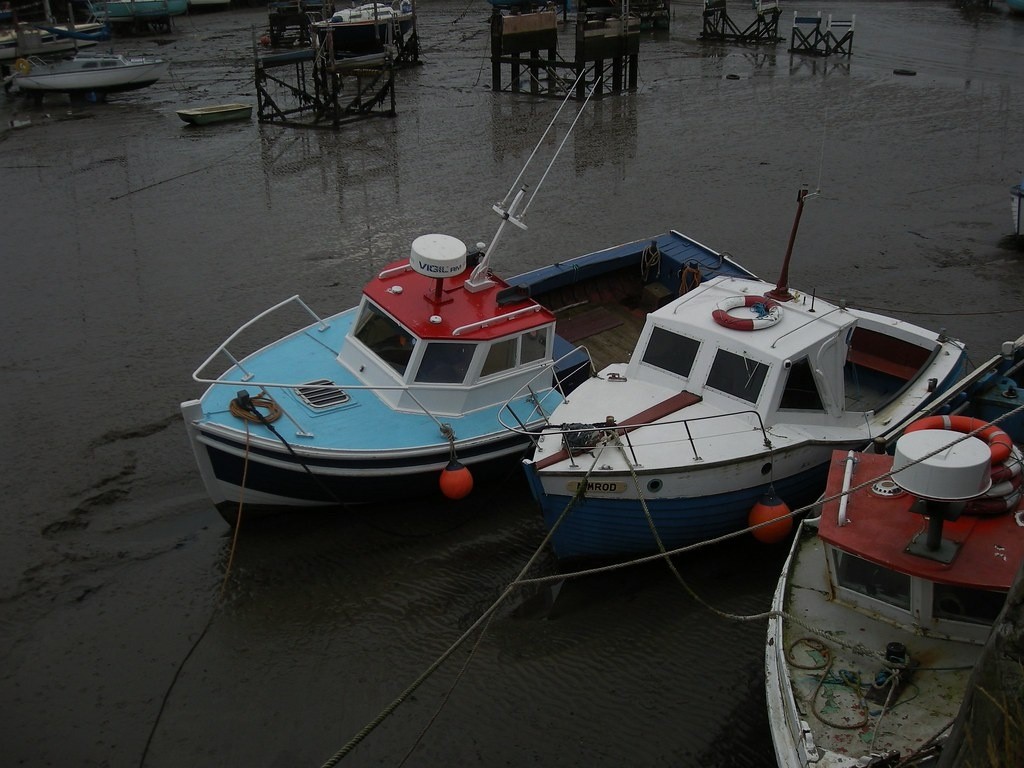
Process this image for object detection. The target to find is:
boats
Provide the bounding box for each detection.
[175,102,254,126]
[495,185,970,554]
[252,0,335,65]
[1009,184,1024,237]
[178,63,769,537]
[0,0,189,98]
[759,331,1024,768]
[304,0,415,73]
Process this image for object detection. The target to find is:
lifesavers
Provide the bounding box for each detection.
[903,416,1024,517]
[712,294,784,330]
